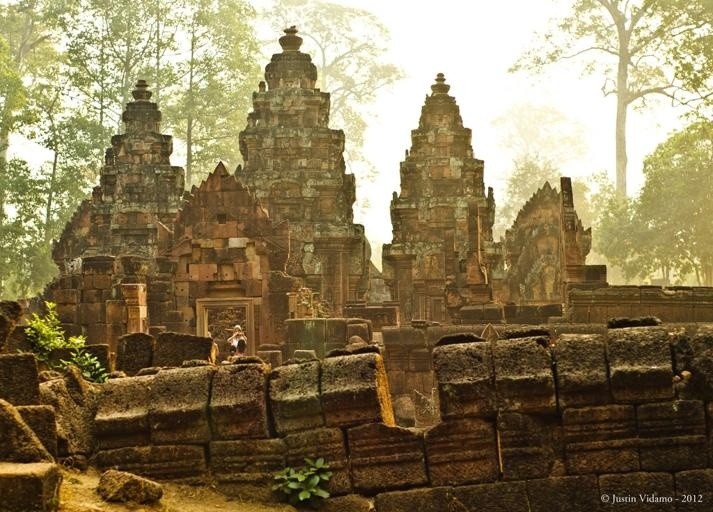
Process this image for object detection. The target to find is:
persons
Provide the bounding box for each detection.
[207,331,219,364]
[226,323,248,348]
